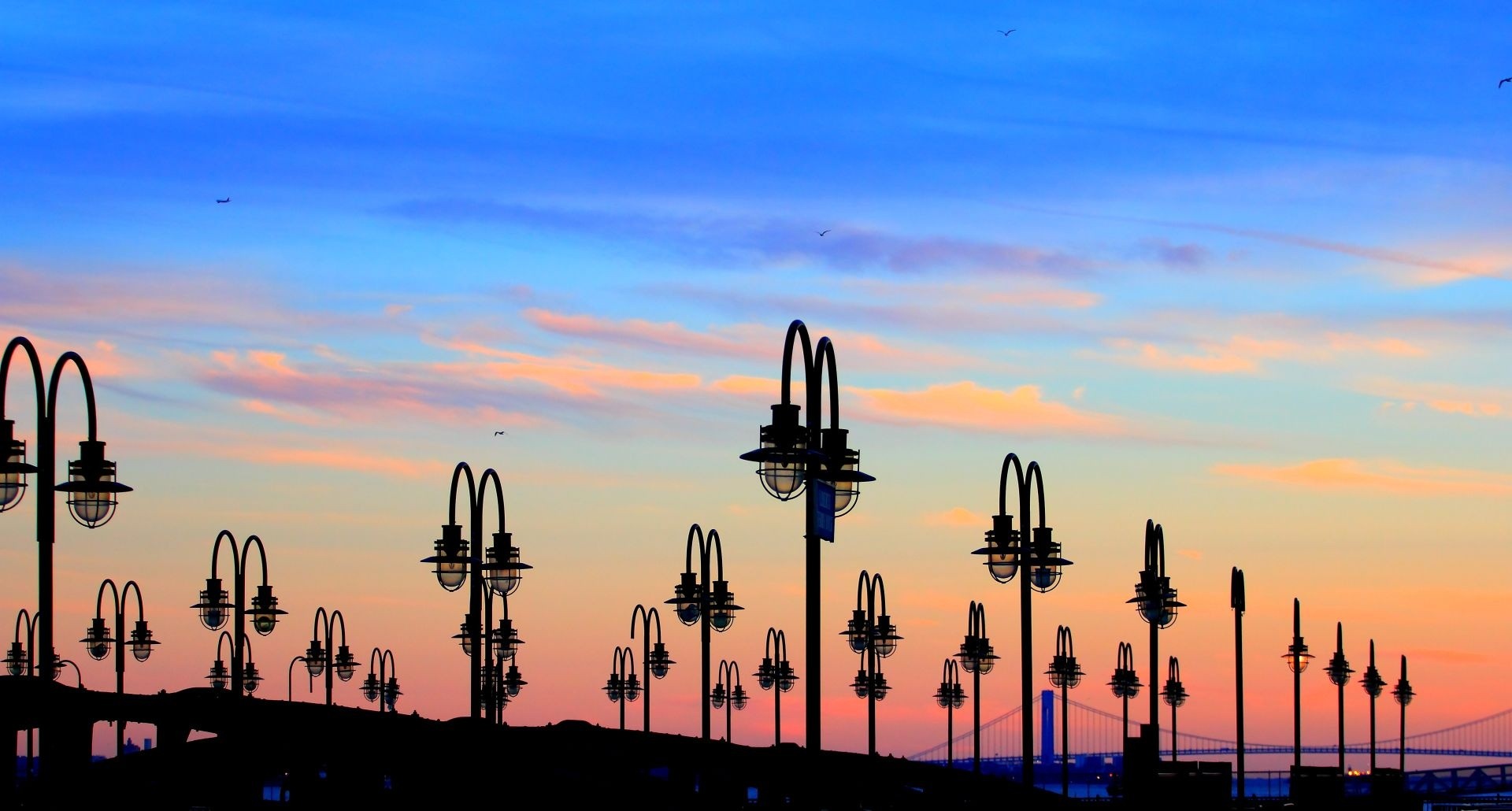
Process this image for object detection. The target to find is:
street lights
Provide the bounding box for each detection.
[1322,620,1365,775]
[838,569,903,761]
[1158,655,1193,767]
[0,604,43,676]
[1,335,133,693]
[629,604,678,730]
[76,579,161,695]
[414,459,528,715]
[1104,642,1149,770]
[659,523,746,746]
[1042,625,1092,794]
[190,530,294,698]
[706,658,751,746]
[933,657,970,768]
[298,605,362,708]
[450,575,536,726]
[730,320,877,775]
[970,450,1075,789]
[1276,595,1317,764]
[1388,653,1417,774]
[1120,518,1189,765]
[601,644,649,729]
[750,627,799,751]
[954,599,1008,778]
[357,649,403,715]
[1360,638,1388,769]
